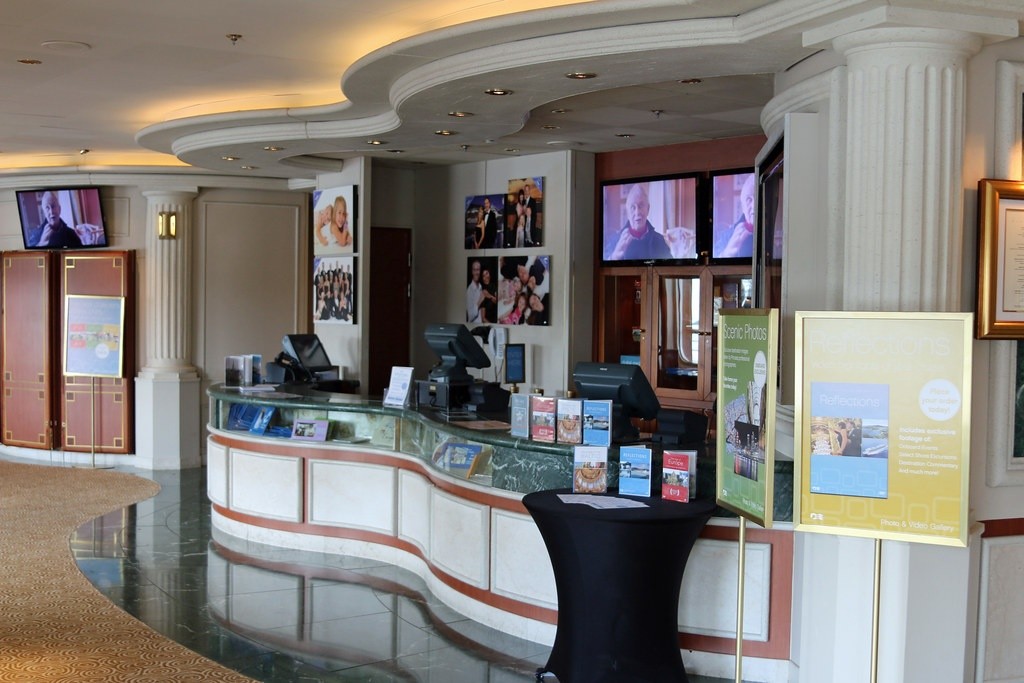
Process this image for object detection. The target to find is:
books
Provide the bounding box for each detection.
[511,393,542,439]
[532,396,556,443]
[619,446,651,497]
[662,450,697,503]
[240,386,275,391]
[557,399,582,444]
[583,400,613,446]
[573,446,607,494]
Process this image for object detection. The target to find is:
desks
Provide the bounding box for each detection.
[522,487,716,683]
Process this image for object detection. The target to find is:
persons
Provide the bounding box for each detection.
[835,421,862,457]
[603,185,687,260]
[515,184,537,248]
[27,191,82,246]
[473,198,497,249]
[498,256,549,326]
[717,174,756,256]
[69,331,120,345]
[316,196,352,247]
[304,424,314,437]
[466,260,497,324]
[314,260,353,321]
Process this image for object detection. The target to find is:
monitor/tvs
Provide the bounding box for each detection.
[573,361,661,421]
[423,323,491,369]
[598,165,758,267]
[771,171,785,267]
[16,188,110,249]
[283,333,332,372]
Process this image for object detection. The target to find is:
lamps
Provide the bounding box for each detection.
[158,211,177,240]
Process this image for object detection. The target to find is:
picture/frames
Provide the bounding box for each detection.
[715,306,780,529]
[973,178,1024,342]
[791,310,974,548]
[63,294,125,379]
